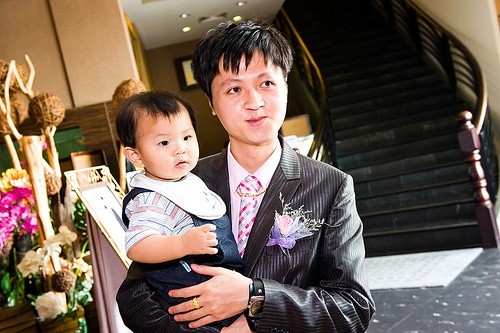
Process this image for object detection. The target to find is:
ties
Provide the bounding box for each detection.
[236,176,264,258]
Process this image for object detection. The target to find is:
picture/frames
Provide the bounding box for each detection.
[62,165,133,269]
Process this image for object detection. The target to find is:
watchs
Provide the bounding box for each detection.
[248,277,266,319]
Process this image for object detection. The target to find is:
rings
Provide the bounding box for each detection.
[192,296,200,309]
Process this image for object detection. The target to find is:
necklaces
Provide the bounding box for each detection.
[234,185,265,196]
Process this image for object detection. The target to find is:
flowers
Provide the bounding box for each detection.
[265,193,324,250]
[0,166,94,322]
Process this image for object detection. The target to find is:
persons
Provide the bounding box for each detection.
[115,18,378,332]
[114,89,253,332]
